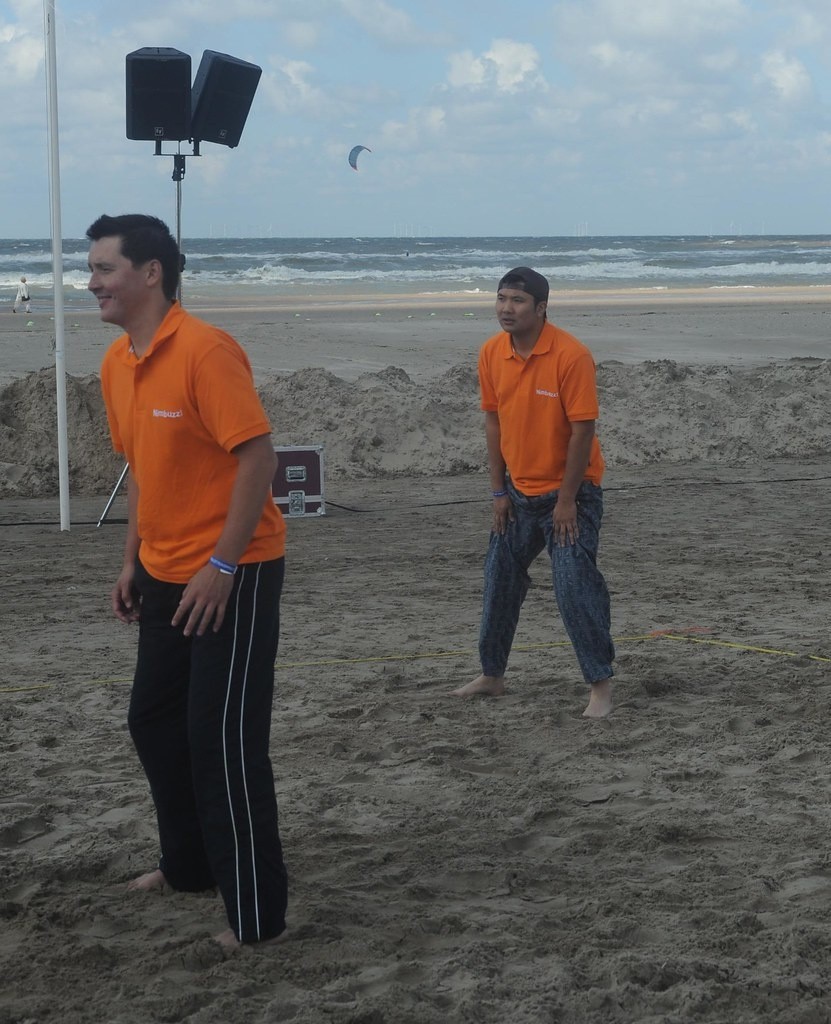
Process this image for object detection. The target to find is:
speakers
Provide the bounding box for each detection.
[124,47,191,142]
[191,49,262,150]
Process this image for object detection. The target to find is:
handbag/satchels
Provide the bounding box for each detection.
[22,296,30,301]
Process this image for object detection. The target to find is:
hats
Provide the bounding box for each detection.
[498,267,549,318]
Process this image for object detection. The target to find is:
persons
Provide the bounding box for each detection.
[86,211,289,943]
[454,266,614,718]
[13,276,33,313]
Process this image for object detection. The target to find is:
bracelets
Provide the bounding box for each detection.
[210,556,237,576]
[493,488,507,496]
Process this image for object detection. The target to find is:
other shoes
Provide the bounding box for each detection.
[13,309,16,313]
[27,311,32,313]
